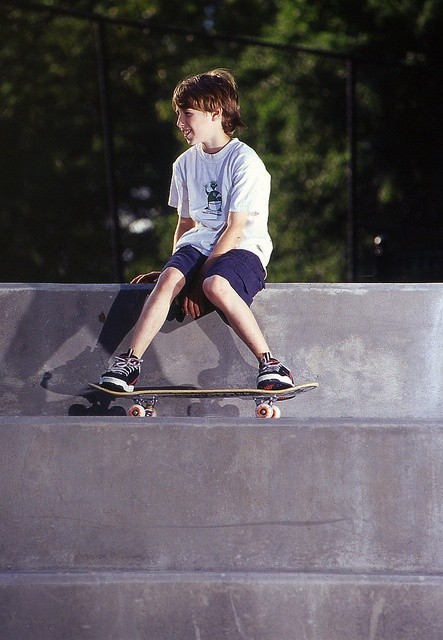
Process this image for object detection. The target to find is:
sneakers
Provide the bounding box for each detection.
[256,351,294,392]
[100,349,144,394]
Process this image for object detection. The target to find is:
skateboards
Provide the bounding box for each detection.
[87,383,318,419]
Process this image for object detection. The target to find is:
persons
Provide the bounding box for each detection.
[99,67,294,392]
